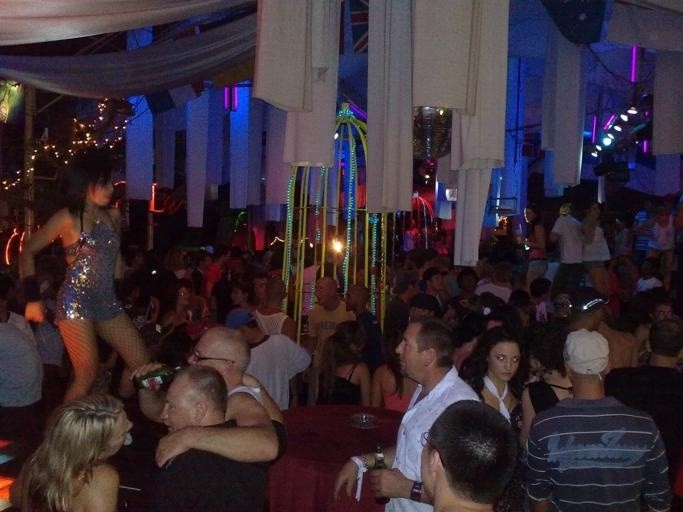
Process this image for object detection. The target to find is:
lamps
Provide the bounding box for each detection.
[591,92,654,158]
[279,100,388,344]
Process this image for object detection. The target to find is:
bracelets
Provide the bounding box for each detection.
[21,273,42,303]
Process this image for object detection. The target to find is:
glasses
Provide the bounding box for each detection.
[420,432,445,468]
[190,348,234,363]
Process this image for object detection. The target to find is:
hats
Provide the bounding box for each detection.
[223,308,254,329]
[562,327,610,376]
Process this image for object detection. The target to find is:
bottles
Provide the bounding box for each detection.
[132,365,193,390]
[372,441,390,506]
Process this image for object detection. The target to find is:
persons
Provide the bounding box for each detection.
[0,194,682,511]
[18,154,148,406]
